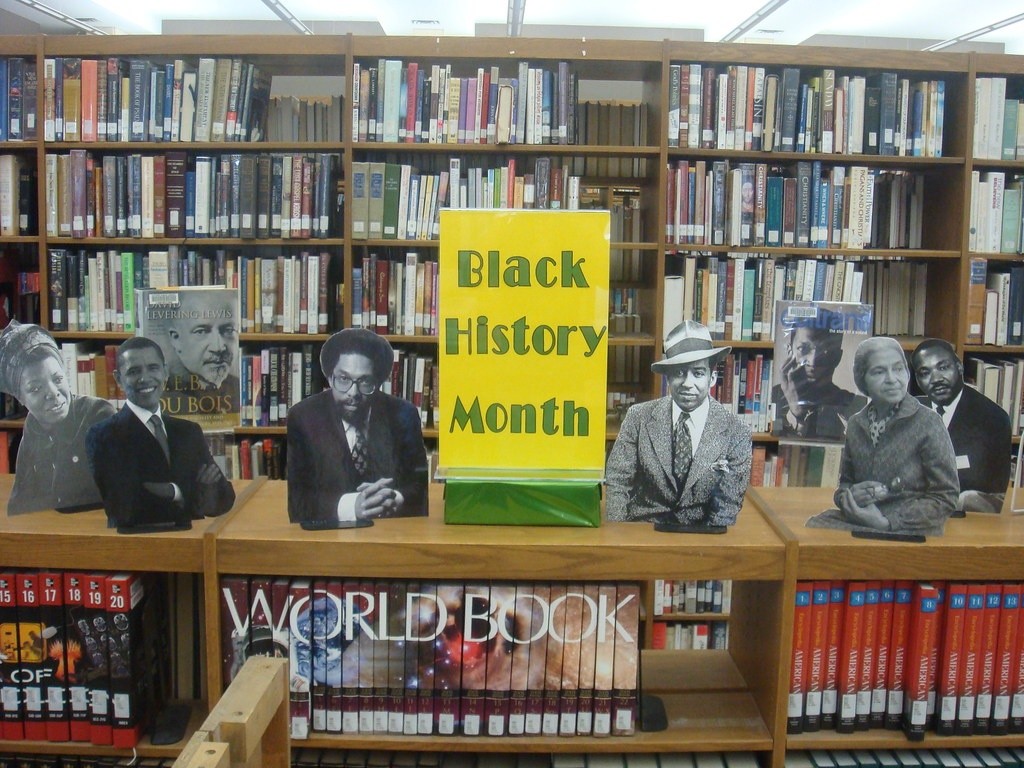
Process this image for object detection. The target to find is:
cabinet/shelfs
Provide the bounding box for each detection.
[0,34,1024,768]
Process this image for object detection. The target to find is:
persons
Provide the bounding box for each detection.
[1,289,1012,535]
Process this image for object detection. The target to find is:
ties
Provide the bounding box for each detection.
[936,405,945,416]
[671,412,693,492]
[150,413,171,462]
[351,422,372,482]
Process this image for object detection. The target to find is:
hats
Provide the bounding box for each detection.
[650,319,734,372]
[1,318,57,398]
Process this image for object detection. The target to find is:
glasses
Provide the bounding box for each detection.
[329,369,380,396]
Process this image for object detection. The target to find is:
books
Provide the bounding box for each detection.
[663,63,950,487]
[353,59,650,432]
[0,571,204,768]
[0,55,41,476]
[963,77,1024,488]
[219,578,766,768]
[784,577,1024,768]
[47,50,353,478]
